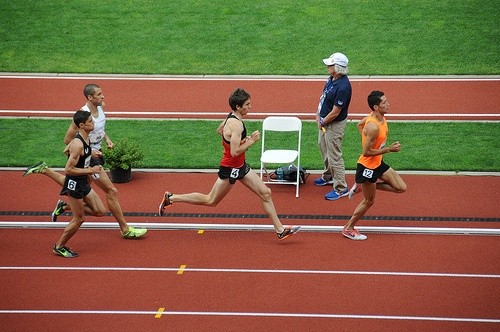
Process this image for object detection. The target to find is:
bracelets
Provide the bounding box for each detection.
[319,119,325,125]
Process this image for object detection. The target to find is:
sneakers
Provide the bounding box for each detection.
[121,227,148,239]
[53,245,78,258]
[325,188,349,200]
[348,182,362,198]
[51,200,67,222]
[314,177,334,185]
[277,226,301,240]
[342,227,367,240]
[22,161,48,176]
[159,192,173,215]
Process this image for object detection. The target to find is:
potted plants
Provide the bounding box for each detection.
[101,138,143,183]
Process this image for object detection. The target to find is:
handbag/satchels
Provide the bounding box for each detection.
[268,163,304,185]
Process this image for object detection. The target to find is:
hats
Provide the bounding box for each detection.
[323,52,349,67]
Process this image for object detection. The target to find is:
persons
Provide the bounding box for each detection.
[342,90,406,240]
[52,110,106,258]
[159,88,302,241]
[314,52,350,201]
[23,84,148,239]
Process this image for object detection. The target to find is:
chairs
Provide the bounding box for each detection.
[260,115,302,198]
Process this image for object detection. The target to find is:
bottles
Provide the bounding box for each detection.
[277,165,283,180]
